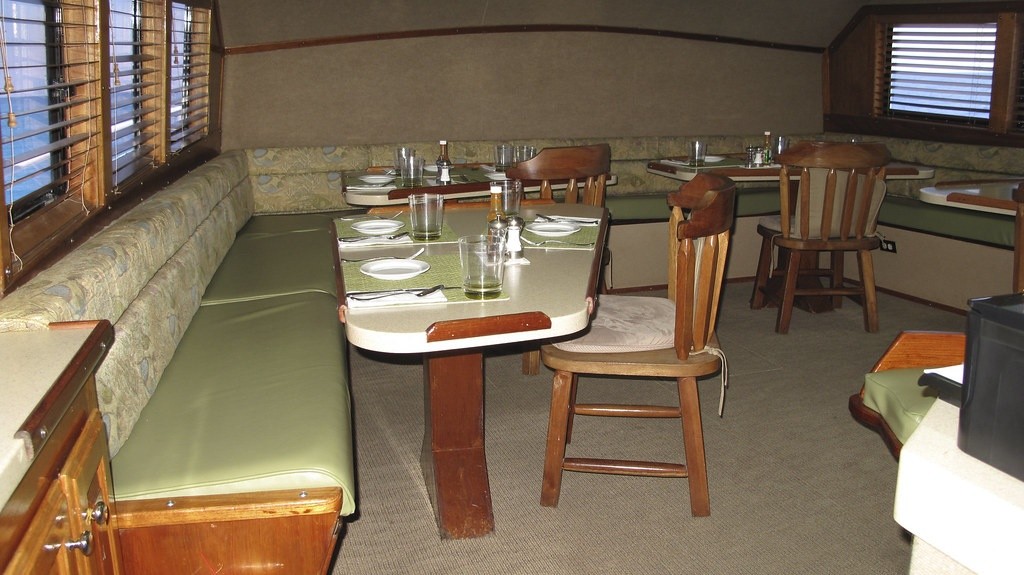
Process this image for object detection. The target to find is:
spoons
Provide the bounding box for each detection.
[520,236,594,246]
[340,211,404,221]
[537,214,599,225]
[343,247,425,264]
[337,231,409,243]
[350,284,443,301]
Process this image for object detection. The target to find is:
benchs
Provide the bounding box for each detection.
[0,132,1024,575]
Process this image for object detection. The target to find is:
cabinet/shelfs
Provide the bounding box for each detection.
[0,372,127,575]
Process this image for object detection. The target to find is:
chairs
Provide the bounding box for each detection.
[750,141,892,334]
[540,171,737,516]
[506,143,612,374]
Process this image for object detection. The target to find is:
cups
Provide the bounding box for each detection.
[393,146,423,188]
[689,140,707,166]
[490,179,522,217]
[408,194,444,241]
[459,234,506,299]
[494,145,537,172]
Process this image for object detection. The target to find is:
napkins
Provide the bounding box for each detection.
[646,153,936,315]
[480,164,496,171]
[346,287,447,309]
[385,168,396,174]
[535,215,601,227]
[336,234,413,247]
[485,171,511,181]
[346,184,397,190]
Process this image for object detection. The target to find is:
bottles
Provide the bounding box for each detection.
[487,186,525,260]
[744,130,789,168]
[436,140,452,184]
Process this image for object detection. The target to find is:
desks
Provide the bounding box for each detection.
[343,163,618,206]
[330,199,610,540]
[918,178,1024,294]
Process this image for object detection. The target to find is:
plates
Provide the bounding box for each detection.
[424,165,455,172]
[485,172,511,180]
[525,222,582,237]
[351,220,431,282]
[695,156,725,161]
[359,174,397,184]
